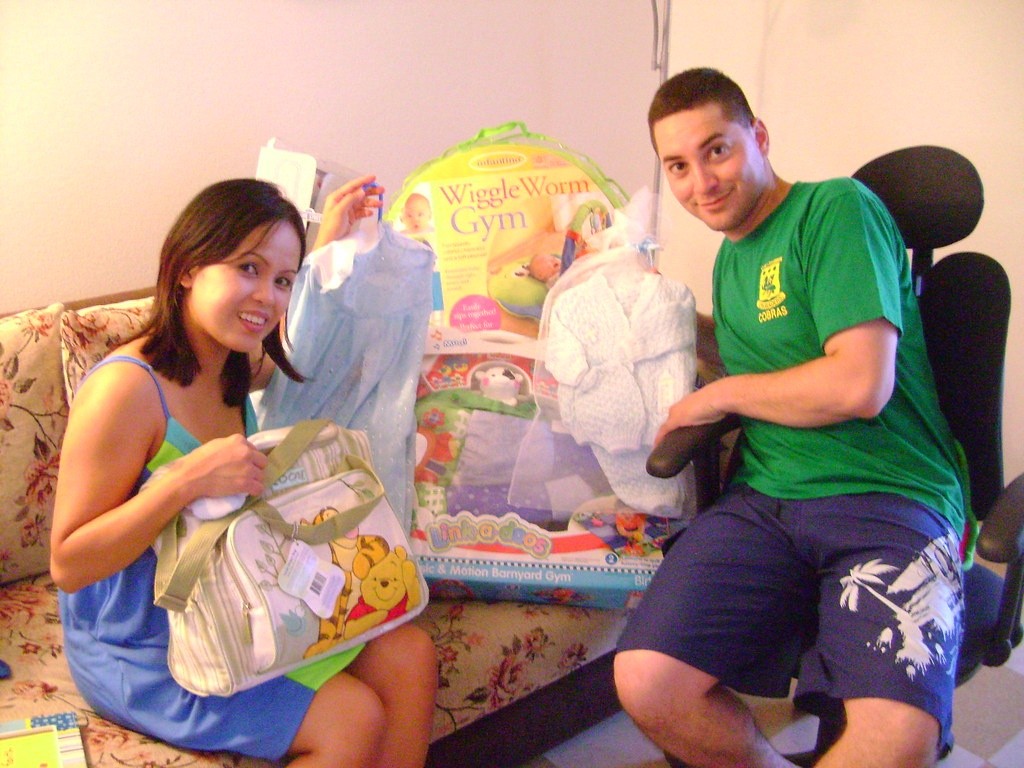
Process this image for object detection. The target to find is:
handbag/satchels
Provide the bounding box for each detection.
[138,418,429,698]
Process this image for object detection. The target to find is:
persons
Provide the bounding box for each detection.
[52,172,437,768]
[530,251,561,290]
[610,67,965,768]
[396,193,436,235]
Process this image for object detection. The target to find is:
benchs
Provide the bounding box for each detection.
[1,263,742,768]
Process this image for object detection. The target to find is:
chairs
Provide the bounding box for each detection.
[647,144,1024,754]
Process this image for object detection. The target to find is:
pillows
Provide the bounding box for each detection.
[55,293,163,410]
[1,298,75,588]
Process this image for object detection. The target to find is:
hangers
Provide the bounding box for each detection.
[350,180,433,270]
